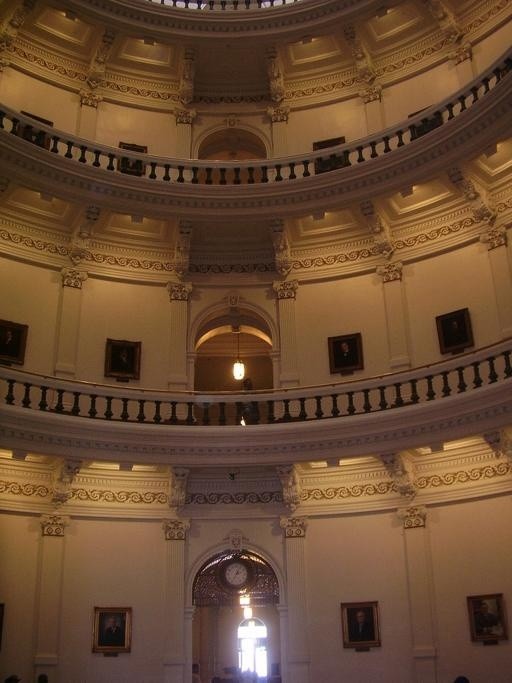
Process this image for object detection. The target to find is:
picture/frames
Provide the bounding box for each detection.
[434,307,474,355]
[327,332,364,375]
[118,141,148,176]
[465,592,509,643]
[0,317,28,366]
[16,109,53,151]
[340,600,381,649]
[92,605,132,653]
[103,337,141,381]
[313,135,350,175]
[408,102,442,141]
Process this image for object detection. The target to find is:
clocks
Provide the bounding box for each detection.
[215,553,258,596]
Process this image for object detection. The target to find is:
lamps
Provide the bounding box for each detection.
[233,334,245,381]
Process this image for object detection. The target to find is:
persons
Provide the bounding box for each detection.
[102,615,125,645]
[2,327,16,355]
[235,377,261,424]
[333,340,357,366]
[351,609,373,641]
[112,343,132,371]
[443,318,468,348]
[476,600,500,634]
